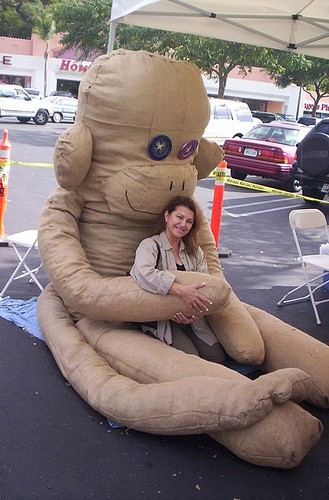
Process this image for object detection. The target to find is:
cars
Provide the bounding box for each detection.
[269,121,307,129]
[49,91,74,99]
[222,123,311,192]
[41,96,78,123]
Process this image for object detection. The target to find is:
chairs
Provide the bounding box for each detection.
[0,228,45,298]
[272,132,283,141]
[276,207,329,326]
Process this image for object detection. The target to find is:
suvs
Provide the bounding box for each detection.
[0,84,54,125]
[253,112,285,123]
[295,117,321,128]
[24,88,49,99]
[289,117,329,206]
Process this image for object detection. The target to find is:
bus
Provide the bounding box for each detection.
[203,97,264,147]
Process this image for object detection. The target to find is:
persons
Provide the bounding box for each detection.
[130,196,228,366]
